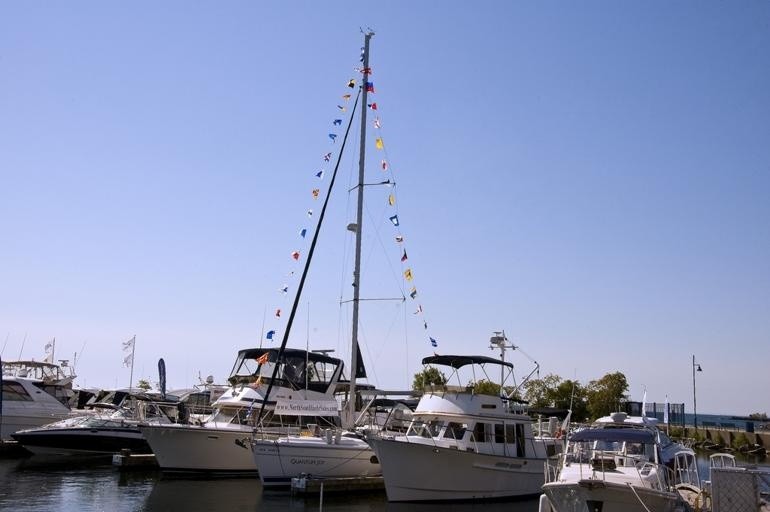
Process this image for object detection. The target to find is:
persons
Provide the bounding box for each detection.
[555,428,567,449]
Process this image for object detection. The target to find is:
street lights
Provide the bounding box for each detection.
[693,355,702,427]
